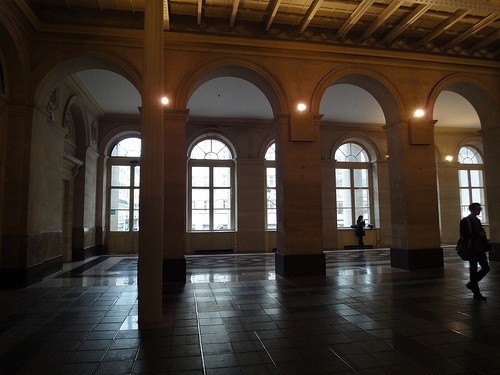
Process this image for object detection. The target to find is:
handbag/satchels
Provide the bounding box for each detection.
[456,234,472,260]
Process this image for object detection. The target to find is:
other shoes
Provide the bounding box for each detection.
[473,292,487,301]
[465,281,474,291]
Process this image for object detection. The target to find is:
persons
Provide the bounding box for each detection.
[357,215,365,249]
[459,203,490,299]
